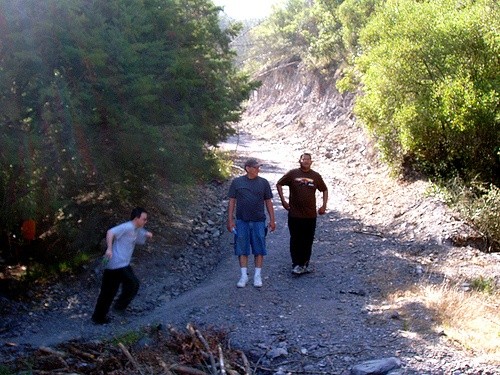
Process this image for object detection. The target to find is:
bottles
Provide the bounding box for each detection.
[226,225,238,235]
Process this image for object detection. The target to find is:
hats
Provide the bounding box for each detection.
[246,158,263,168]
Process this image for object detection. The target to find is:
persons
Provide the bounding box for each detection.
[276,153,328,274]
[92,206,152,324]
[227,158,276,287]
[21,217,39,272]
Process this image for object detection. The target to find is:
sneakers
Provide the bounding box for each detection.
[254,275,262,287]
[291,265,310,274]
[237,275,248,287]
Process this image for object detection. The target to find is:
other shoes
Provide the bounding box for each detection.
[92,316,113,323]
[112,305,136,315]
